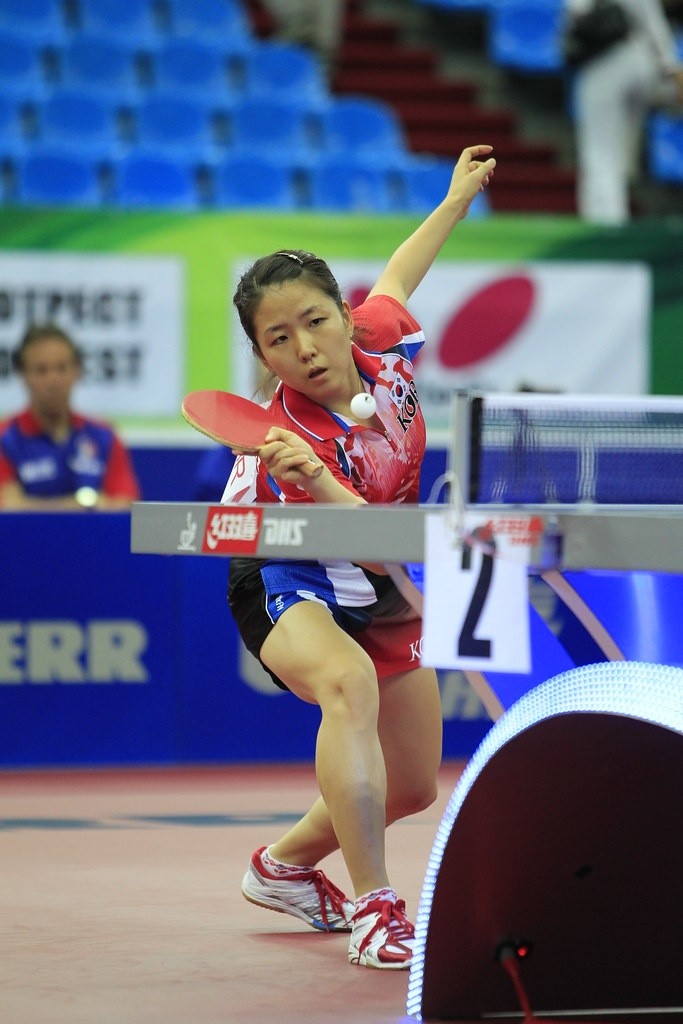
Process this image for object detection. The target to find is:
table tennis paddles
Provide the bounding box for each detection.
[181,388,324,480]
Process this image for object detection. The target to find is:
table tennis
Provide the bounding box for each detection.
[349,392,378,420]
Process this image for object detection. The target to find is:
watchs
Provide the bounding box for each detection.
[226,144,496,969]
[73,485,98,512]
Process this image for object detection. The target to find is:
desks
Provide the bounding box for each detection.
[130,389,683,569]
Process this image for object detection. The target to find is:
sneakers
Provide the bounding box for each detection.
[348,899,415,971]
[241,845,354,932]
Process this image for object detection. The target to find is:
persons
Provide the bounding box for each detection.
[559,1,683,226]
[0,325,140,513]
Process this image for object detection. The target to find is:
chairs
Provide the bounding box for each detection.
[430,0,683,182]
[0,0,488,216]
